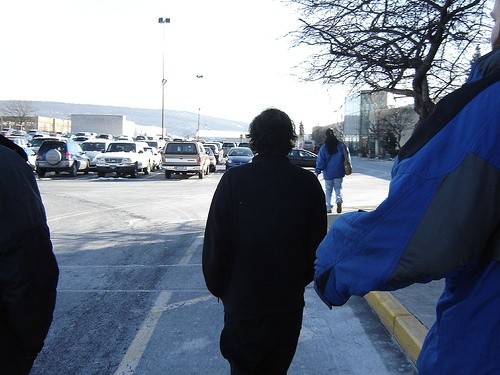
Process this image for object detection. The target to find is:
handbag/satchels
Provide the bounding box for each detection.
[342,143,353,175]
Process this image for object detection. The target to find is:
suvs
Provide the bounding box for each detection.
[36,138,90,176]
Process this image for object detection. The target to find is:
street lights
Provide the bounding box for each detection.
[196,75,204,132]
[158,17,170,140]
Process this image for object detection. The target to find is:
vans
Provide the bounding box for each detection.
[302,140,320,155]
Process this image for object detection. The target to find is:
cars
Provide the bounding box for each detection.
[80,134,182,179]
[202,142,237,173]
[288,148,317,167]
[0,128,97,173]
[224,143,255,170]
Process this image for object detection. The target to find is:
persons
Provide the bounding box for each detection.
[0,133,60,375]
[201,107,327,375]
[313,0,500,375]
[314,129,348,213]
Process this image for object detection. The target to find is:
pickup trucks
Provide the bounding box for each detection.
[162,141,210,179]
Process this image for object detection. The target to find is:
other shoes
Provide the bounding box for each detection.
[337,202,342,213]
[327,209,332,213]
